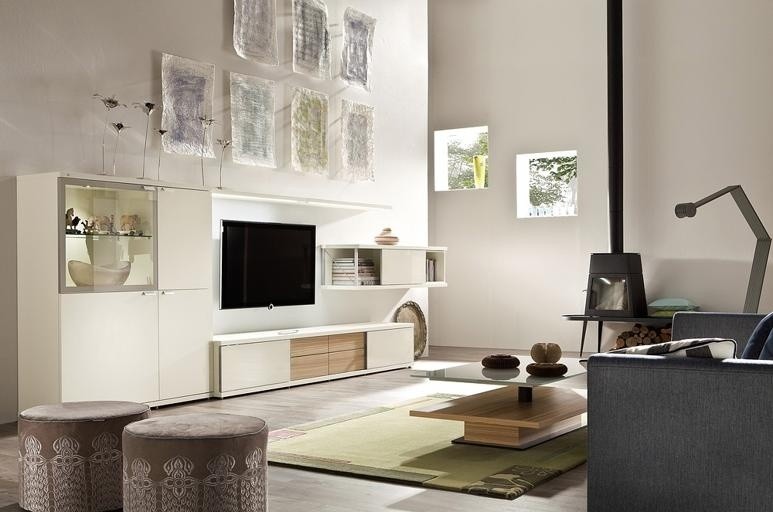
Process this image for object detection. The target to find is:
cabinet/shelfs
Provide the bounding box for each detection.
[317,242,449,291]
[209,321,414,401]
[14,172,216,413]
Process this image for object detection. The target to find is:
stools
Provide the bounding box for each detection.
[16,398,150,511]
[119,412,269,511]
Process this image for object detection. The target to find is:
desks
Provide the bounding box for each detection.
[406,354,588,450]
[560,311,676,358]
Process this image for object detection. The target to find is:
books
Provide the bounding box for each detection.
[426,258,435,282]
[332,257,380,285]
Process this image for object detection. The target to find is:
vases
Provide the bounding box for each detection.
[471,154,487,190]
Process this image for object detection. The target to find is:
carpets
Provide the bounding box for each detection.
[263,392,590,500]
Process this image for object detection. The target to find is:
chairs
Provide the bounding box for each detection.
[584,310,773,511]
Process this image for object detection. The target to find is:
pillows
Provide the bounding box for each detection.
[578,336,738,378]
[643,296,701,310]
[648,310,679,319]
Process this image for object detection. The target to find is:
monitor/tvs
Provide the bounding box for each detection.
[218,218,316,311]
